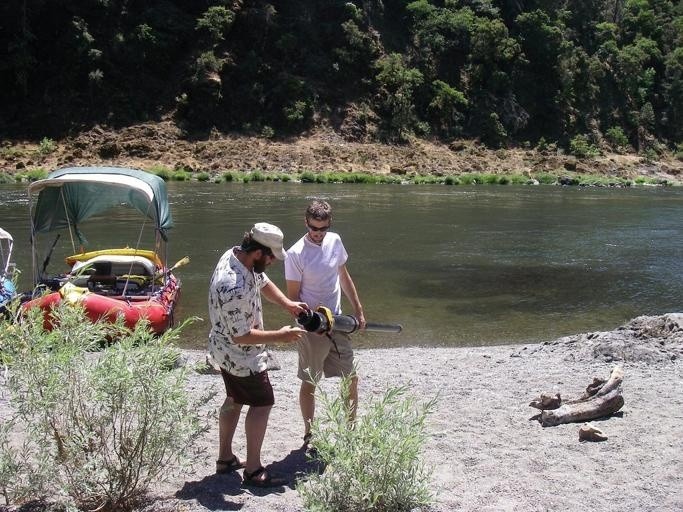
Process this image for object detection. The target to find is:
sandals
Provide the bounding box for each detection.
[242,466,289,487]
[214,454,245,474]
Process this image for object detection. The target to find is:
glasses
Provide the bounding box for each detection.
[307,223,329,232]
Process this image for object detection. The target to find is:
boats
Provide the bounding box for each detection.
[0,166,181,341]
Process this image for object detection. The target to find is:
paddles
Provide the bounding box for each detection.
[138,254,191,291]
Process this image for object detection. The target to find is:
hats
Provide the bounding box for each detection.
[248,222,288,262]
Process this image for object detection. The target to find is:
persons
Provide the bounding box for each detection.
[283,199,367,458]
[206,220,312,487]
